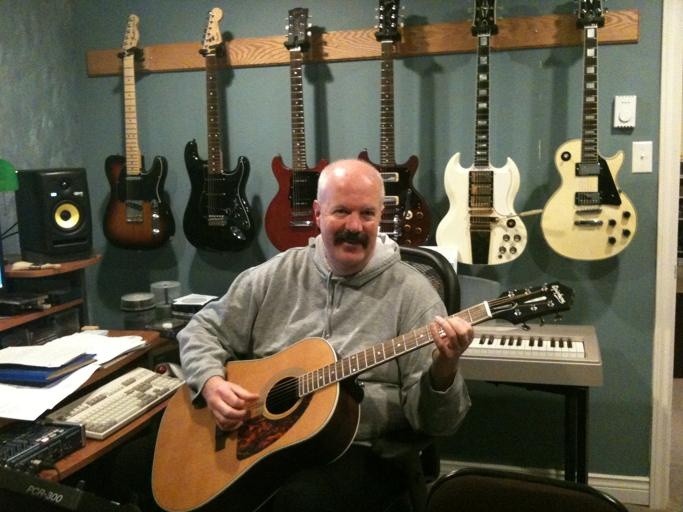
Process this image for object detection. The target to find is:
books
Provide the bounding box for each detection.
[0,345,98,386]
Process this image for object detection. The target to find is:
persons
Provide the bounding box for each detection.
[175,158,473,511]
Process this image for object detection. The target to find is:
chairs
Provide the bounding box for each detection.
[424,464,627,511]
[397,243,460,482]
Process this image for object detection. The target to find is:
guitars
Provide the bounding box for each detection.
[182,6,256,252]
[266,7,330,251]
[151,283,572,511]
[356,1,431,248]
[436,1,528,265]
[103,15,175,249]
[541,1,638,261]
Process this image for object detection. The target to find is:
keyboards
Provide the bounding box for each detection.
[44,365,187,441]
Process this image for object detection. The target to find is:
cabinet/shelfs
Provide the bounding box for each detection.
[1,254,170,511]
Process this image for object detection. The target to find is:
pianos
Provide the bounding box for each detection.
[457,325,603,388]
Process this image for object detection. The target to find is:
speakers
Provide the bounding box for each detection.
[16,167,93,263]
